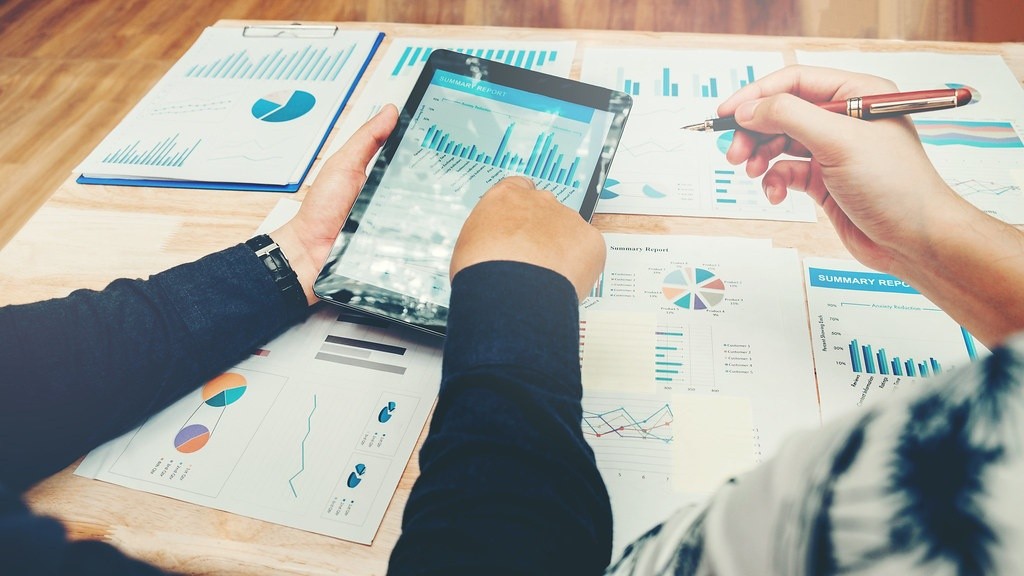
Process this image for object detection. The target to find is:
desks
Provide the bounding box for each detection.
[0,18,1024,576]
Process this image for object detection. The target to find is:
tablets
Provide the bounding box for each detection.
[312,49,633,338]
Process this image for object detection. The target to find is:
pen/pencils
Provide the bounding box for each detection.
[682,88,973,132]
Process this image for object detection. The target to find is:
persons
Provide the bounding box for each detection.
[606,65,1024,576]
[0,103,614,576]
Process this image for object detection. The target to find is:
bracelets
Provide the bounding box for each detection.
[245,234,308,322]
